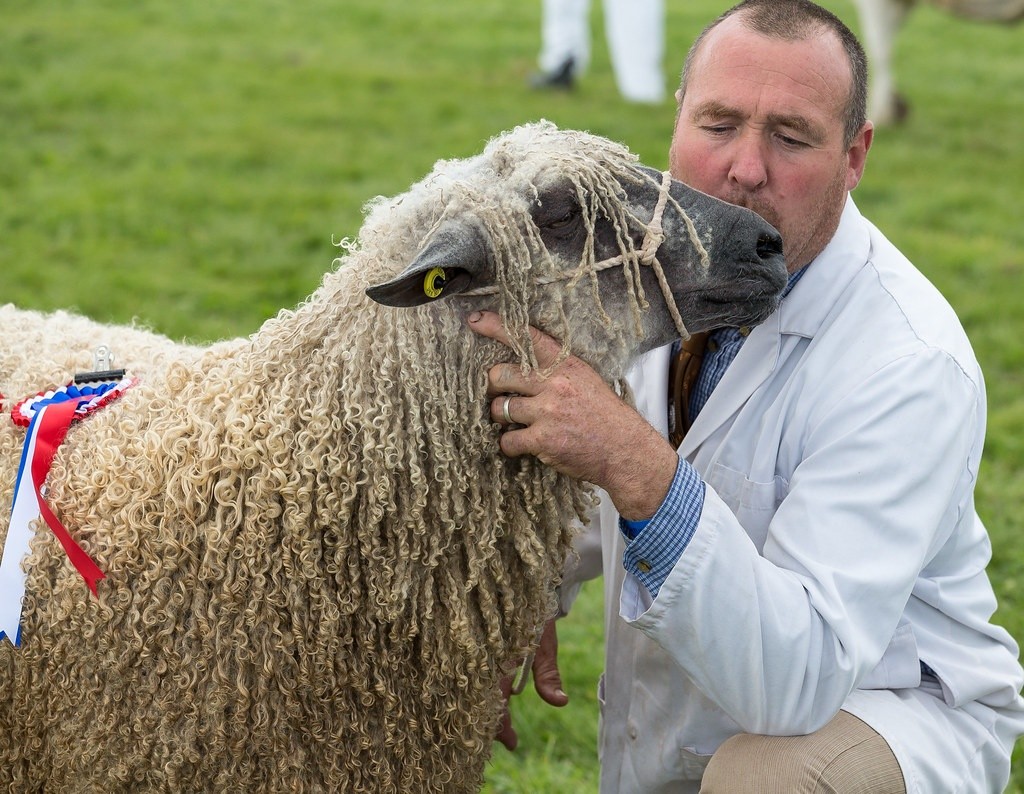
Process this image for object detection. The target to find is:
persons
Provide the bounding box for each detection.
[474,0,1024,794]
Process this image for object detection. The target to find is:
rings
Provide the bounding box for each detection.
[502,395,516,424]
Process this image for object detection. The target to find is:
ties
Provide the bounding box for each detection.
[667,330,718,449]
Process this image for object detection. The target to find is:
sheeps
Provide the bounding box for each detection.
[0,119,787,794]
